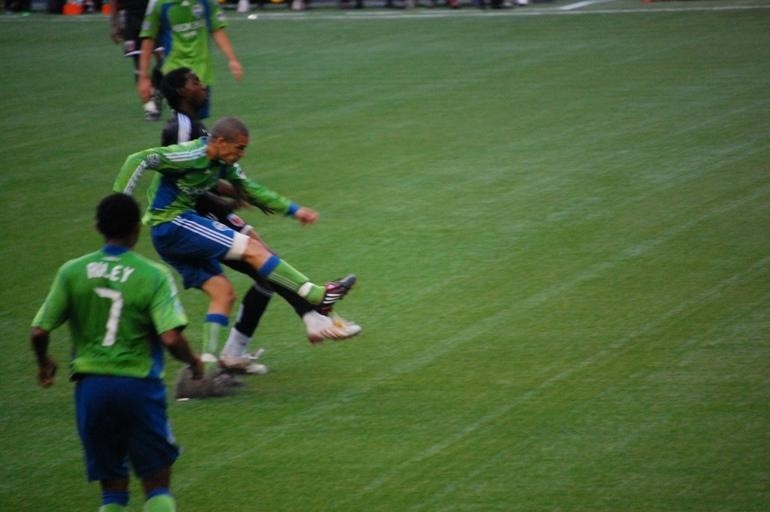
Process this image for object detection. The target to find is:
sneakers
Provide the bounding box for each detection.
[143,88,163,121]
[202,350,269,387]
[305,274,361,343]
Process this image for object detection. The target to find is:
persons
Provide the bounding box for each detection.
[28,192,202,511]
[111,67,365,400]
[111,0,242,120]
